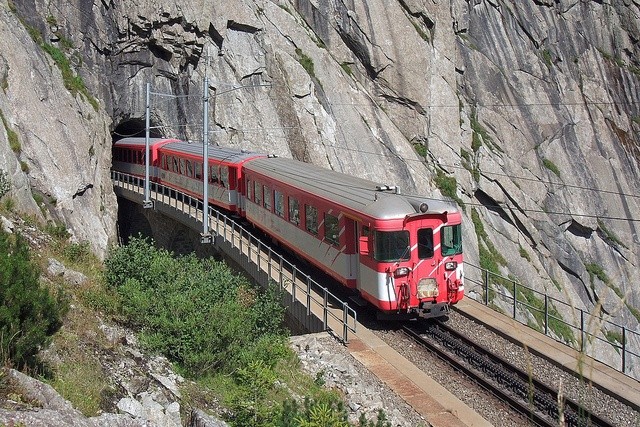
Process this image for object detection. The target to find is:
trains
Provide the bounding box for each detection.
[112,137,464,322]
